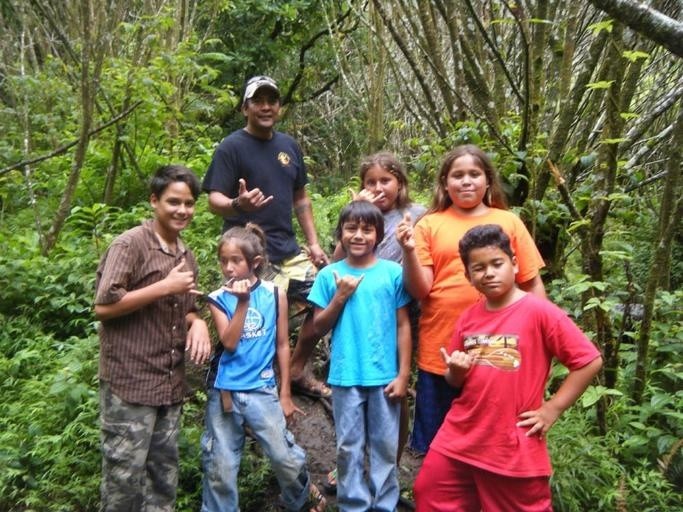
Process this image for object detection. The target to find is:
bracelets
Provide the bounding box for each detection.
[231,197,247,217]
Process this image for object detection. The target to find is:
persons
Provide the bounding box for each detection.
[306,199,413,511]
[393,142,549,505]
[198,74,333,400]
[320,151,429,490]
[195,220,328,512]
[409,223,606,511]
[91,162,213,511]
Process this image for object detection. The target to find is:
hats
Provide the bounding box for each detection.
[242,76,279,104]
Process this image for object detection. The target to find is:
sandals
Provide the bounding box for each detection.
[307,467,338,511]
[290,371,332,398]
